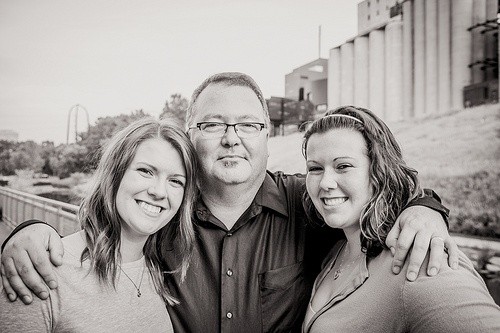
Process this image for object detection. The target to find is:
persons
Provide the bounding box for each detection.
[300,103,500,332]
[2,71,460,332]
[2,118,202,333]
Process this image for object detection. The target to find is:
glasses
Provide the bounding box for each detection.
[188,120,266,137]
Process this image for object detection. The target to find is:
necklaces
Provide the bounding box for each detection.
[333,241,363,280]
[114,260,148,297]
